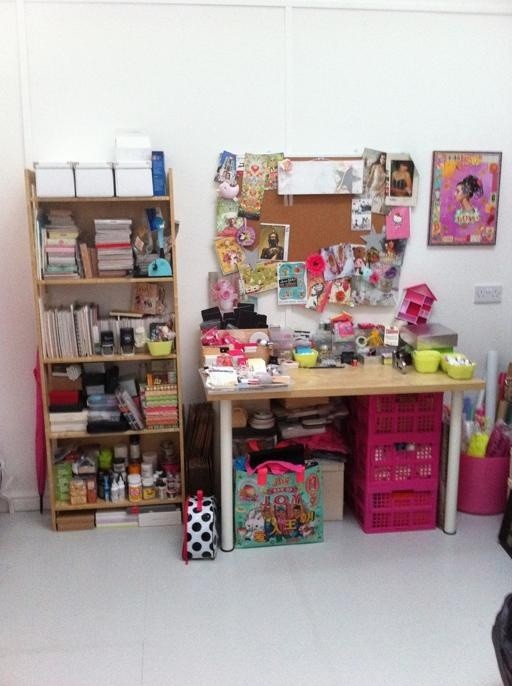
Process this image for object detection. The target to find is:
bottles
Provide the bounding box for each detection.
[99,436,167,504]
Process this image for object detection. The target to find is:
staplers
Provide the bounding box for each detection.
[120,328,136,357]
[102,330,115,357]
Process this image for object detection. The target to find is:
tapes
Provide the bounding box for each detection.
[292,349,318,367]
[280,360,299,371]
[249,332,270,345]
[355,336,367,348]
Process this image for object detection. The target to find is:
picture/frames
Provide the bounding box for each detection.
[427,150,503,248]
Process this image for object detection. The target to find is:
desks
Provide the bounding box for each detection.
[195,352,489,551]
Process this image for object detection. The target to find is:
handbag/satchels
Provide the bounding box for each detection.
[234,439,326,551]
[180,489,219,564]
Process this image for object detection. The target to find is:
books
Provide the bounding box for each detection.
[47,360,179,433]
[34,207,171,280]
[38,282,177,359]
[56,503,182,531]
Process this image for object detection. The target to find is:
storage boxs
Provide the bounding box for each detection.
[318,455,346,522]
[32,160,154,198]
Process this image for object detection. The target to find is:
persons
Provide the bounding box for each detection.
[367,152,386,197]
[391,161,412,196]
[260,232,284,260]
[453,176,485,225]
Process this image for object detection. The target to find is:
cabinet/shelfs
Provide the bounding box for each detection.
[27,167,185,530]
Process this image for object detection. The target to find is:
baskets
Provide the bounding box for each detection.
[342,391,446,533]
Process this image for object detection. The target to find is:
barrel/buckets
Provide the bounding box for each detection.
[458,452,510,515]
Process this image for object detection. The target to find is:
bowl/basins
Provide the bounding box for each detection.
[412,350,476,380]
[147,341,171,356]
[293,348,319,368]
